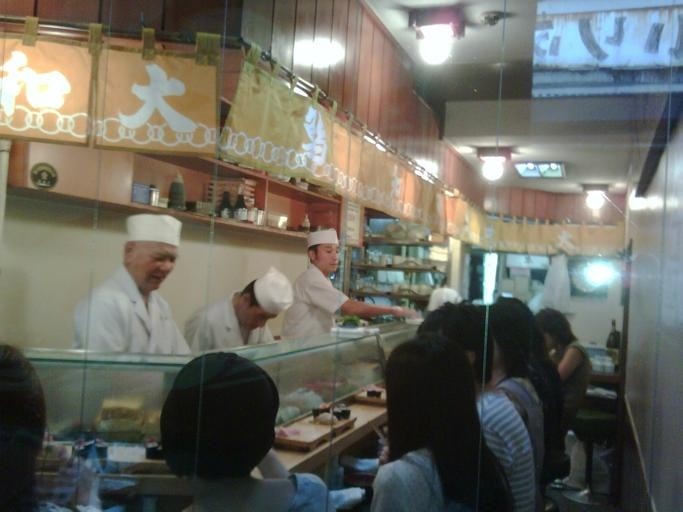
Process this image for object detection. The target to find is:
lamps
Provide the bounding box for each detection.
[581,183,608,211]
[407,5,465,68]
[475,145,510,181]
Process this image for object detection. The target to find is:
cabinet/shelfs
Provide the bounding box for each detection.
[341,233,447,298]
[4,94,342,245]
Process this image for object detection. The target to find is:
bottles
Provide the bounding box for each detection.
[605,318,621,371]
[218,186,246,221]
[351,274,374,290]
[168,171,187,211]
[300,213,311,234]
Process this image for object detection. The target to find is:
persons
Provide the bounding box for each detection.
[73,212,192,443]
[285,227,418,375]
[0,344,104,512]
[184,266,294,384]
[160,354,336,511]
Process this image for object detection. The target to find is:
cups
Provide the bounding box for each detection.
[82,445,110,459]
[390,284,434,296]
[392,255,401,265]
[380,254,388,267]
[149,188,160,208]
[365,391,383,398]
[312,407,351,420]
[246,207,264,226]
[144,447,168,462]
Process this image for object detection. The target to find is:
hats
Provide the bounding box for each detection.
[123,213,182,247]
[254,265,294,315]
[309,227,339,249]
